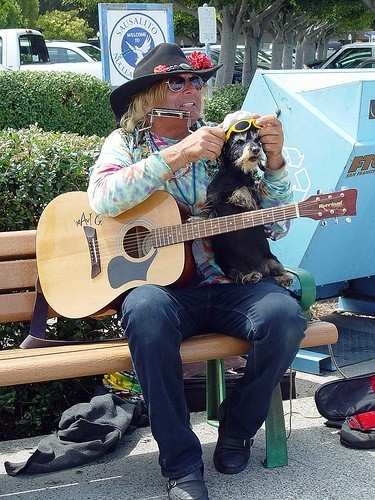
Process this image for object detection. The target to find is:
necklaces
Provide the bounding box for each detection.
[149,129,196,214]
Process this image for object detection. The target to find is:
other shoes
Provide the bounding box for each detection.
[166,462,209,500]
[212,431,255,474]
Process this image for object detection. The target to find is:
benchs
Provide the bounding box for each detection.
[0,230,338,468]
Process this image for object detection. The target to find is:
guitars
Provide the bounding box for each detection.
[35,188,357,321]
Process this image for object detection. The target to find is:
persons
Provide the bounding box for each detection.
[88,43,306,500]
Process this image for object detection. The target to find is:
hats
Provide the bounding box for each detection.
[109,42,224,120]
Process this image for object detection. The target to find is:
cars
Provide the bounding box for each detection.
[178,43,298,70]
[43,42,102,60]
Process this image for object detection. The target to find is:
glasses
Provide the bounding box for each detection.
[224,118,264,143]
[161,75,204,93]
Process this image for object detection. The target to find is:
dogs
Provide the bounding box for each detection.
[190,111,297,289]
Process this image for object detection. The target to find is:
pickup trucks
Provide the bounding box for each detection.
[0,28,107,83]
